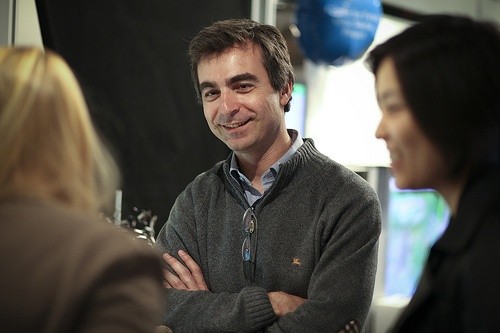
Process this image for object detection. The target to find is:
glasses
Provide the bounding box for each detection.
[242,206,258,265]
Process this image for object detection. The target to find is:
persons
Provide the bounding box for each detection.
[154,19,382,333]
[0,46,166,333]
[363,12,500,333]
